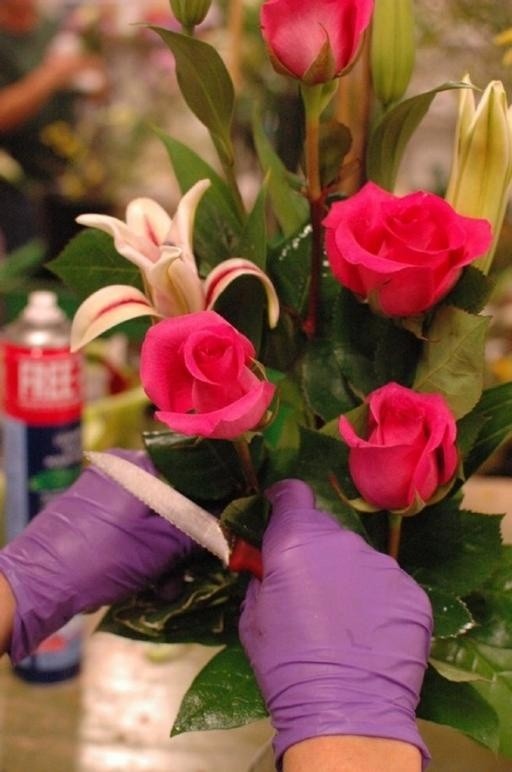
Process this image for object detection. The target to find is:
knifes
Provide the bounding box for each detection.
[82,448,264,583]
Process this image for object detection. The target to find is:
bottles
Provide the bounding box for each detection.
[1,292,89,682]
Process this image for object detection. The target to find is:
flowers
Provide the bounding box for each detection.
[39,0,512,772]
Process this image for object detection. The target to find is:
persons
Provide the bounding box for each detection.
[0,453,435,770]
[0,2,108,244]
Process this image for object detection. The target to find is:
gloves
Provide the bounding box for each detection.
[0,446,207,667]
[236,475,439,772]
